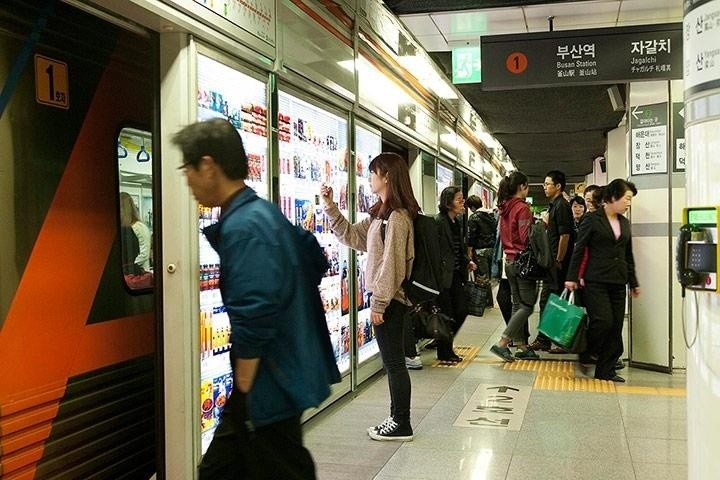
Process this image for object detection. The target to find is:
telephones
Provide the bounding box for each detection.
[677,223,717,287]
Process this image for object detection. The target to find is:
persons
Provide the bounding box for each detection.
[320,153,423,442]
[120,193,151,271]
[169,117,342,480]
[405,171,638,382]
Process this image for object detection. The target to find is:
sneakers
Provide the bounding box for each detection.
[611,375,625,382]
[529,341,564,354]
[490,343,513,362]
[368,415,415,442]
[406,356,424,369]
[515,349,537,361]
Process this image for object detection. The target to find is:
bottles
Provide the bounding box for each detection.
[352,116,381,368]
[196,85,274,368]
[274,85,351,369]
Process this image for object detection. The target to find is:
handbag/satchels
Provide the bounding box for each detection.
[514,222,551,279]
[409,305,457,341]
[538,285,590,350]
[467,262,488,316]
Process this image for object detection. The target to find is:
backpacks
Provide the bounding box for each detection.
[407,209,455,304]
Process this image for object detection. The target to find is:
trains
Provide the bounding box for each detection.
[2,0,519,480]
